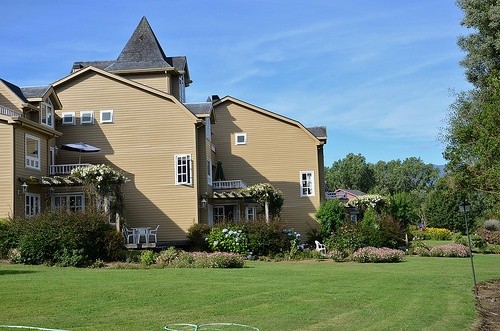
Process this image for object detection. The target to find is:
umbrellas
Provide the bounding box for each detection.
[215,160,226,181]
[60,142,100,163]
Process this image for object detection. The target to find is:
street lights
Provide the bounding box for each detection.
[458,197,477,290]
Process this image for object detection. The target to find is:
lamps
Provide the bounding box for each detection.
[199,198,206,209]
[21,181,29,193]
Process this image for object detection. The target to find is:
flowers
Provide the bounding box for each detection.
[244,182,284,215]
[70,164,131,196]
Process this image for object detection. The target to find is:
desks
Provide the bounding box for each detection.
[130,228,150,243]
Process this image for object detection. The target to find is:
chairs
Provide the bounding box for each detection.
[315,240,326,255]
[125,225,159,243]
